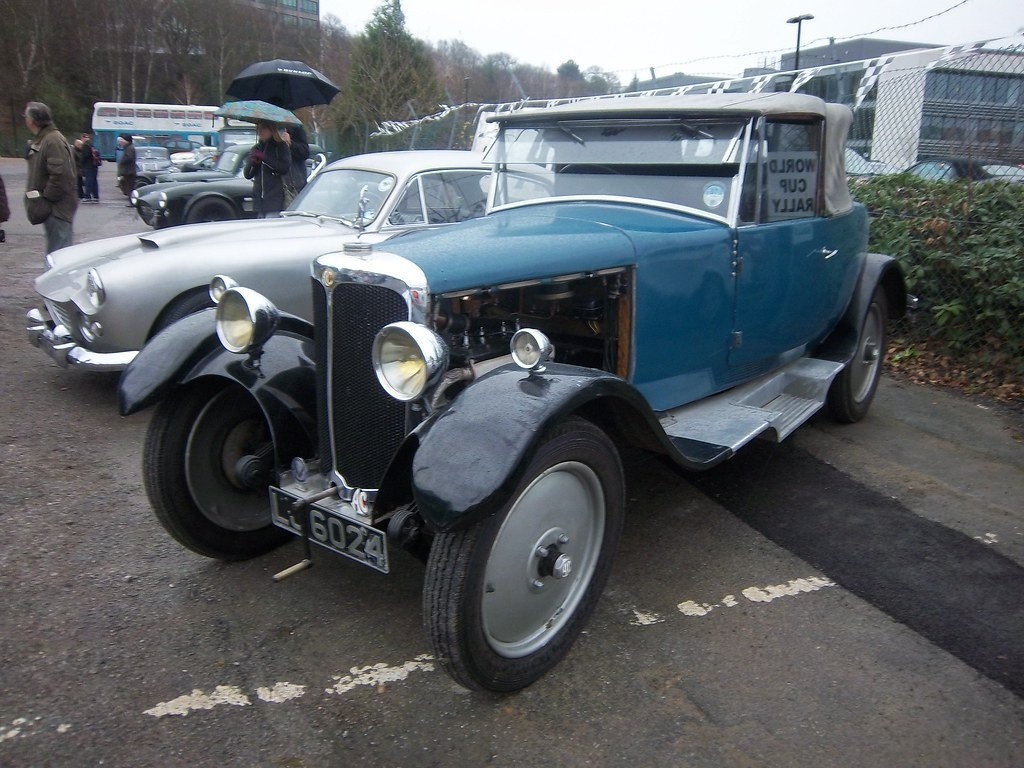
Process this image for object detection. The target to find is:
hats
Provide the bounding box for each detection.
[117,134,133,143]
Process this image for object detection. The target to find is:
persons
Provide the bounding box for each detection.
[70,138,85,198]
[276,114,310,212]
[114,131,140,208]
[244,120,292,219]
[80,133,99,204]
[23,101,77,256]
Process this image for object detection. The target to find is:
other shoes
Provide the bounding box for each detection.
[81,198,99,203]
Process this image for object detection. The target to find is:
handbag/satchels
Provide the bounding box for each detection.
[284,179,299,211]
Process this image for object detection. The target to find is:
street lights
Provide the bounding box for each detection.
[787,14,814,70]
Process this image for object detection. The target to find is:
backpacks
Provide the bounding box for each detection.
[84,142,102,167]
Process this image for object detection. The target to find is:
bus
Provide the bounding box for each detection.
[92,100,225,162]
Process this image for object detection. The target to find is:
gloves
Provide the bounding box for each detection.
[247,147,264,163]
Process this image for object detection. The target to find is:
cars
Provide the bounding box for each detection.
[841,147,879,179]
[130,145,323,230]
[898,157,1023,184]
[131,152,223,189]
[114,85,909,697]
[156,146,255,185]
[23,149,560,382]
[170,147,215,165]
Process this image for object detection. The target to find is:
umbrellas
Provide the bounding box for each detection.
[210,100,304,151]
[225,59,340,113]
